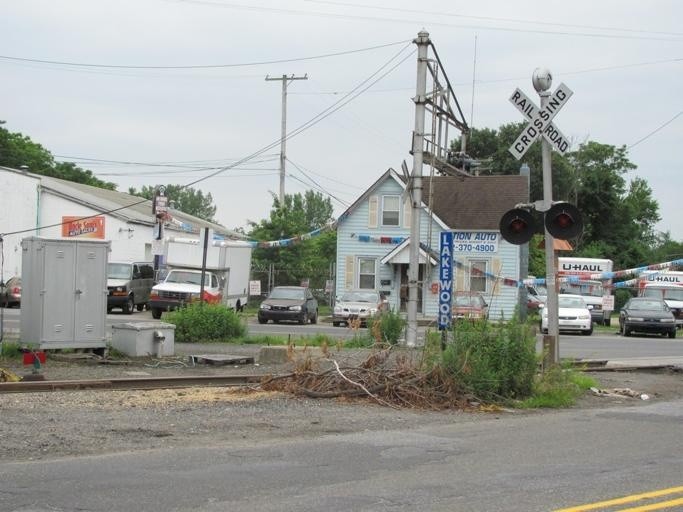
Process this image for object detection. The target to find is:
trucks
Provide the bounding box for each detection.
[640,272,683,328]
[556,257,615,326]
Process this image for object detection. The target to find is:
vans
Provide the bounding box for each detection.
[107,260,154,313]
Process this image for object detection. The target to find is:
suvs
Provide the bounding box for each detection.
[149,269,220,318]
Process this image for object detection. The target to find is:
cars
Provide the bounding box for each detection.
[257,285,318,325]
[2,278,21,306]
[332,290,390,328]
[619,298,676,339]
[451,292,488,329]
[526,276,593,336]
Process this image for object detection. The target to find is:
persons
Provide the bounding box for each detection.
[376,294,390,312]
